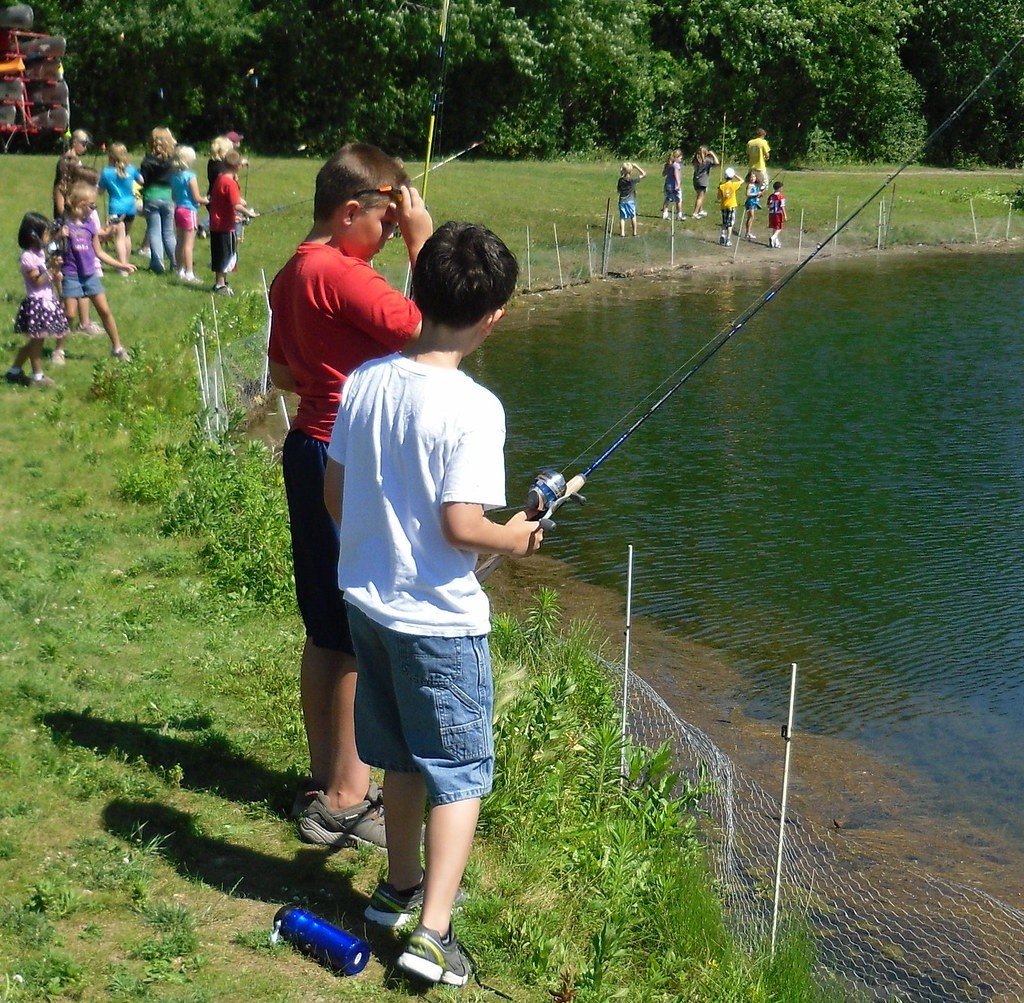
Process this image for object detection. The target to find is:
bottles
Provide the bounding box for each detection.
[273,903,371,976]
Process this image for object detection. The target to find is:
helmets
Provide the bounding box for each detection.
[724,168,735,178]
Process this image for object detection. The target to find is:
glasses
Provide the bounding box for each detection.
[83,200,97,212]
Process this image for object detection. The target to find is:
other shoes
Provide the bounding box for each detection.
[212,282,235,299]
[676,215,686,221]
[768,236,782,249]
[177,269,204,287]
[726,241,733,247]
[756,203,763,209]
[693,213,701,220]
[699,211,707,217]
[719,235,725,245]
[663,213,671,221]
[746,233,756,239]
[115,266,130,277]
[138,247,151,259]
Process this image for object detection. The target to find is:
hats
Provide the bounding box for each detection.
[227,131,243,143]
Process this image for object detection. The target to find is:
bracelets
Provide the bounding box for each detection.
[43,267,57,281]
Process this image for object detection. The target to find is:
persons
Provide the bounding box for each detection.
[98,141,144,276]
[660,149,686,222]
[266,142,436,855]
[170,145,210,286]
[138,211,152,258]
[140,127,197,275]
[323,222,545,987]
[717,167,745,248]
[69,166,119,336]
[53,129,94,220]
[743,172,763,240]
[53,155,81,220]
[616,162,646,238]
[691,146,720,220]
[744,128,770,199]
[49,183,138,366]
[5,211,71,387]
[206,132,261,297]
[766,181,787,247]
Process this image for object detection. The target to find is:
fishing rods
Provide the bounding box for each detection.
[760,160,790,193]
[412,138,483,182]
[717,111,727,190]
[200,157,277,205]
[93,145,100,167]
[101,142,110,251]
[400,0,456,301]
[240,147,253,244]
[477,32,1024,587]
[237,197,314,226]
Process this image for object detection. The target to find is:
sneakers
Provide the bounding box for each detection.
[290,776,428,855]
[51,349,66,367]
[111,348,133,363]
[6,370,29,386]
[76,319,104,338]
[397,919,512,1000]
[364,866,470,927]
[28,374,55,392]
